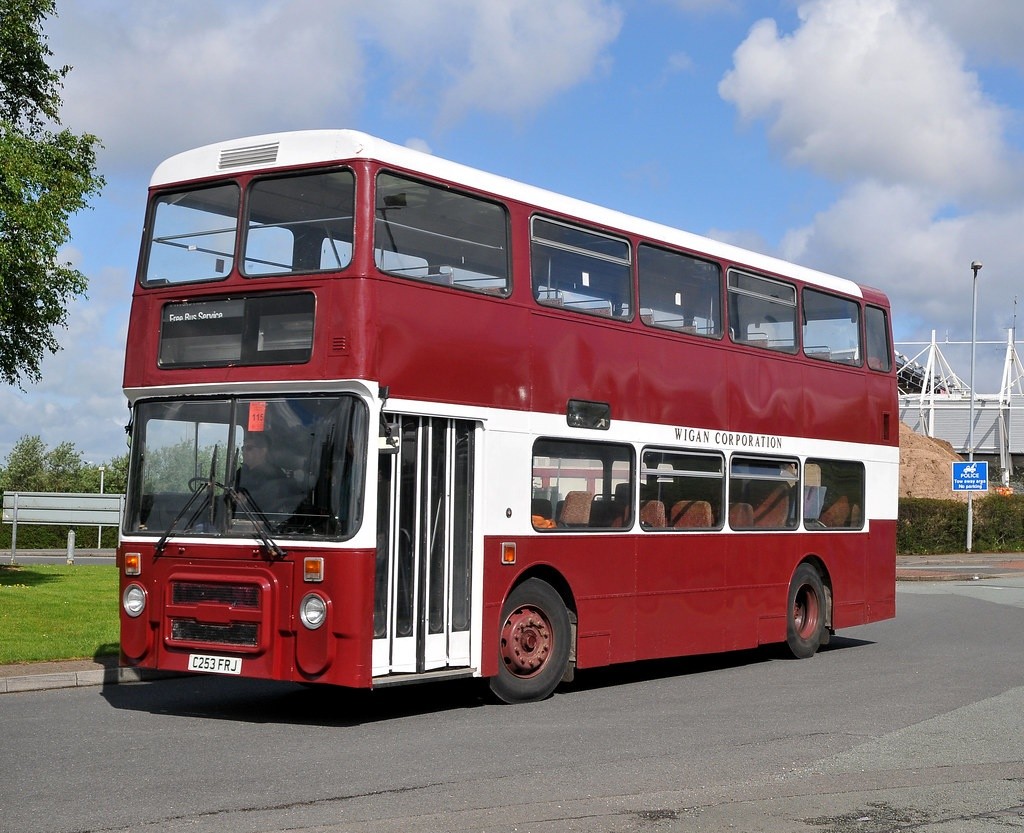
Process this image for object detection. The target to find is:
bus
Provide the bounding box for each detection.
[116,130,900,705]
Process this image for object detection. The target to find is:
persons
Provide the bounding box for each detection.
[235,432,289,493]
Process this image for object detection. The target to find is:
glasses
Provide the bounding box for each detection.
[241,445,260,451]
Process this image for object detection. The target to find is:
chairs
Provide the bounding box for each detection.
[384,264,883,371]
[532,480,862,529]
